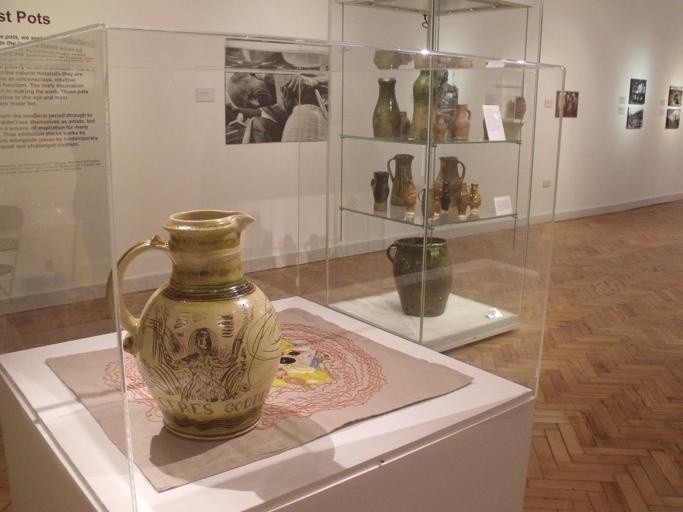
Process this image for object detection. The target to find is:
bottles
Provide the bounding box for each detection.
[372,69,471,143]
[417,178,481,218]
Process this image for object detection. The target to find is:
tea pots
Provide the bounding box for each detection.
[387,154,418,207]
[105,209,282,442]
[435,156,466,193]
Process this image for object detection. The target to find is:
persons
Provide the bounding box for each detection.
[562,92,576,118]
[626,105,643,128]
[630,78,645,103]
[226,73,327,143]
